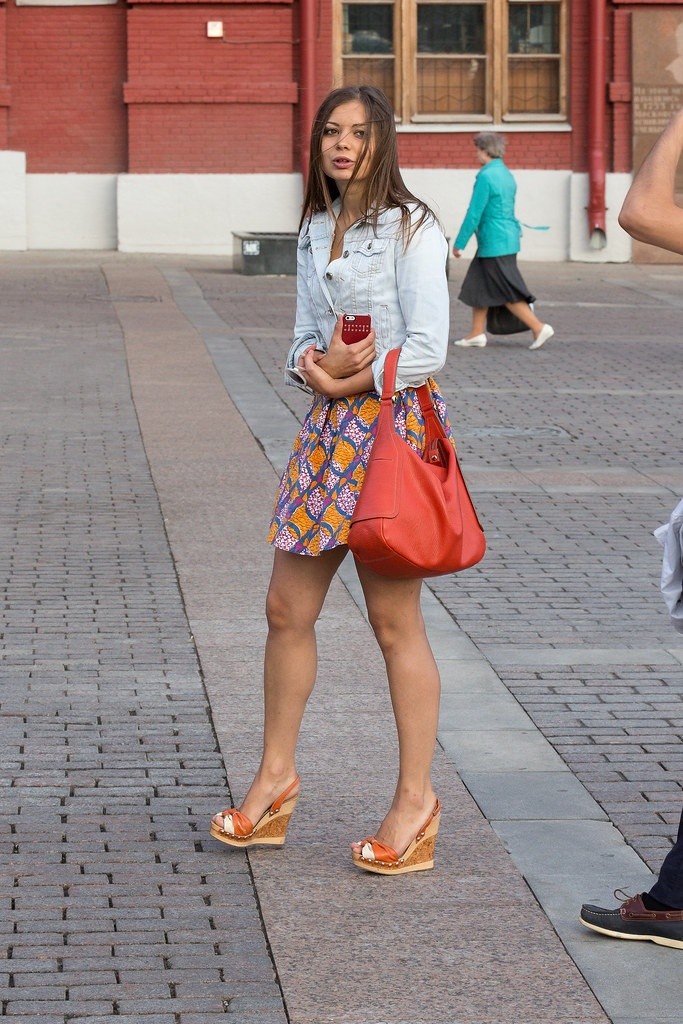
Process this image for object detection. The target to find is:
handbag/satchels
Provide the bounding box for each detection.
[487,303,535,335]
[347,348,486,580]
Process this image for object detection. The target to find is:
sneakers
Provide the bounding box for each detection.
[578,888,683,949]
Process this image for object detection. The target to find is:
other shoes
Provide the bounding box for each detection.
[529,324,554,349]
[454,334,487,347]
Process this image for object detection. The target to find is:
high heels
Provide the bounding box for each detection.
[351,798,440,875]
[209,775,300,849]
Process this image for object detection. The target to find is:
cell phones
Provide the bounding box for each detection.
[342,313,371,346]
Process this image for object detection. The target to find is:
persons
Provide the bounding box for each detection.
[211,87,450,872]
[453,132,555,351]
[578,109,683,948]
